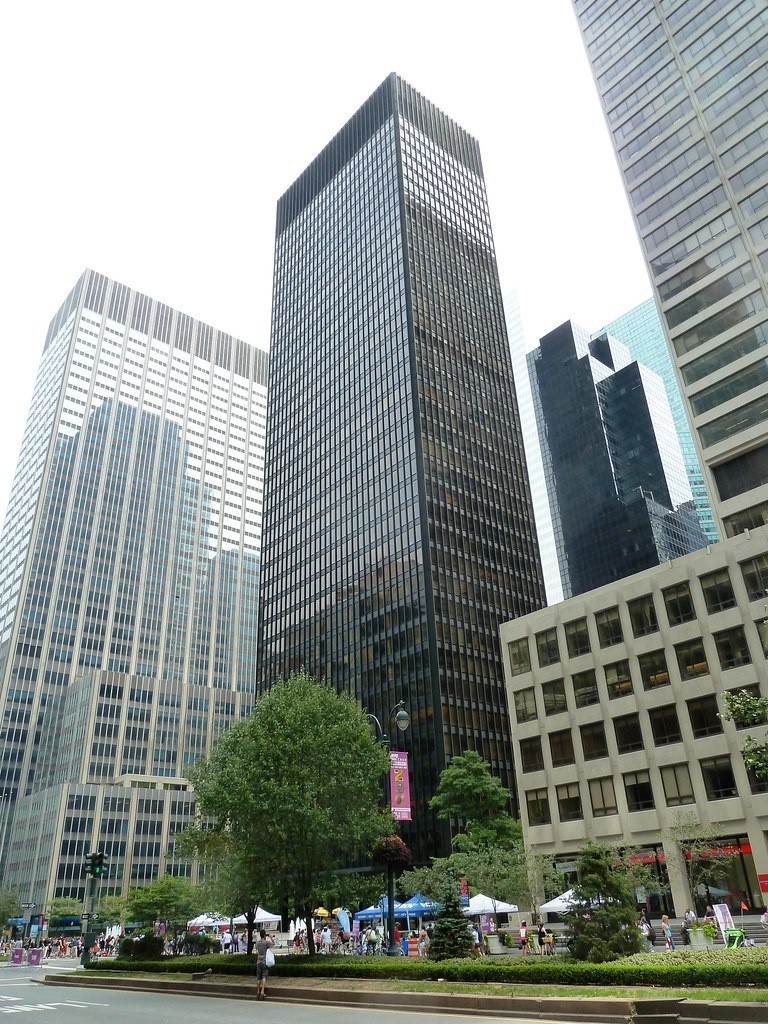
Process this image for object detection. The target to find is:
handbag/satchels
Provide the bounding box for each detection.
[266,949,275,967]
[367,936,377,942]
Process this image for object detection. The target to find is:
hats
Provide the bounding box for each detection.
[339,926,344,930]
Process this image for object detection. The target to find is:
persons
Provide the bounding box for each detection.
[764,910,768,928]
[160,928,278,956]
[518,919,531,957]
[537,921,577,956]
[0,930,140,959]
[255,929,275,1000]
[638,906,717,953]
[292,922,433,960]
[466,918,494,958]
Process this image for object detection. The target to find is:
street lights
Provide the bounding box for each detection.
[365,699,412,959]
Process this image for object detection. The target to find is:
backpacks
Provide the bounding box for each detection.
[343,932,350,940]
[645,924,656,941]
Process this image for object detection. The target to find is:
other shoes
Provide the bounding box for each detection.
[257,993,268,997]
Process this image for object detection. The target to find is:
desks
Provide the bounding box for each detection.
[555,937,572,954]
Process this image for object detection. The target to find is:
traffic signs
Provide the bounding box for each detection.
[21,903,36,909]
[82,913,100,919]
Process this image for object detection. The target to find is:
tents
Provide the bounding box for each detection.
[539,885,621,914]
[187,904,282,955]
[461,894,518,917]
[354,890,445,939]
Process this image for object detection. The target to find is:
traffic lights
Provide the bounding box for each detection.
[101,853,108,880]
[94,852,101,880]
[84,852,93,875]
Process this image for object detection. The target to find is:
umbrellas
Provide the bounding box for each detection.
[312,906,351,930]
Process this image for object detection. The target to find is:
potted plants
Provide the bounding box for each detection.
[454,848,571,955]
[647,810,741,950]
